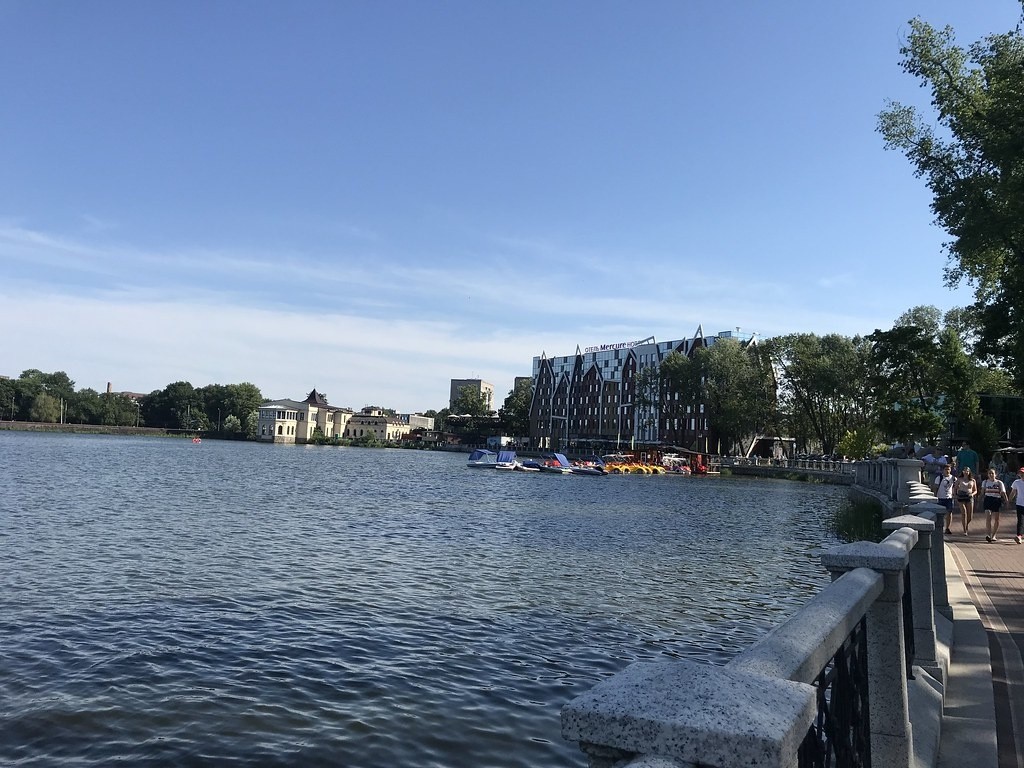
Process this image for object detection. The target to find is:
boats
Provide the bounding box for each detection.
[466,449,691,476]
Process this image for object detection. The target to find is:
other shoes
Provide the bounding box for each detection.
[964,532,968,536]
[945,529,952,534]
[991,537,997,541]
[1015,536,1022,544]
[986,535,992,542]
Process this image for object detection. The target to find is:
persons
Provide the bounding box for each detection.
[833,450,843,467]
[989,452,1010,489]
[981,467,1007,542]
[922,448,946,492]
[954,438,979,480]
[934,463,955,534]
[955,465,978,536]
[1008,466,1024,543]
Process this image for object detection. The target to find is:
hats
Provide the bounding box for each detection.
[1019,467,1024,473]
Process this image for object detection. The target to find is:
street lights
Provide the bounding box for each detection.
[65,400,67,423]
[12,397,14,415]
[946,412,957,463]
[136,403,139,426]
[218,408,220,431]
